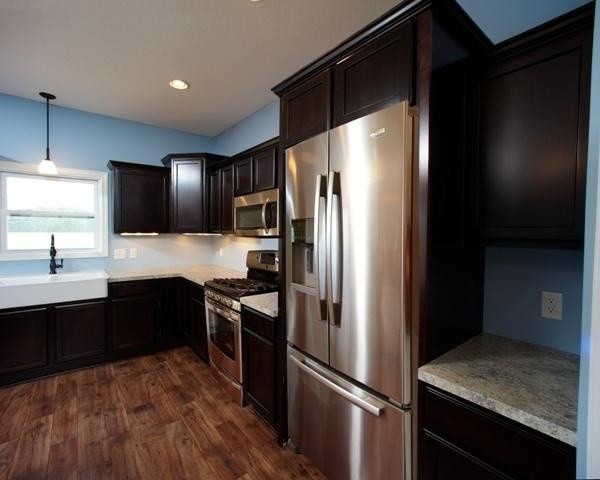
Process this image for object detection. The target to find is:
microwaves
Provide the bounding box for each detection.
[232,188,282,237]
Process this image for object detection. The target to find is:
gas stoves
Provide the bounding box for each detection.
[203,277,280,313]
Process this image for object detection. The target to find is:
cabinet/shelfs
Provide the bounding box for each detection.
[0,297,111,387]
[486,0,596,250]
[273,0,495,150]
[417,378,576,480]
[105,134,281,238]
[241,304,287,448]
[108,276,209,367]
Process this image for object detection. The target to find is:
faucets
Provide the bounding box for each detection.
[48,234,63,273]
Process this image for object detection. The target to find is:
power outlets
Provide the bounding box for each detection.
[540,291,563,319]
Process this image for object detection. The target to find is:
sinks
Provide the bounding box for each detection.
[0,273,107,309]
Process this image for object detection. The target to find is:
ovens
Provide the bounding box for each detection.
[204,295,243,408]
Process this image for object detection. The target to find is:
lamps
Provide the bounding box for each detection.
[38,92,61,175]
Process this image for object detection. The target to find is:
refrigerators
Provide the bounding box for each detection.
[286,101,422,480]
